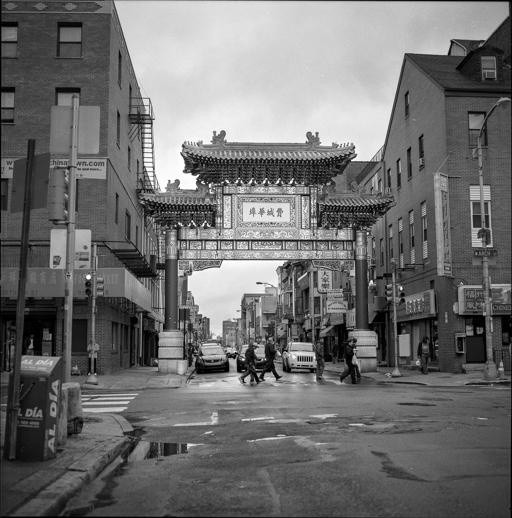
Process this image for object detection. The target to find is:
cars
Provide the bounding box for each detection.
[195,343,237,374]
[282,341,324,373]
[235,344,271,373]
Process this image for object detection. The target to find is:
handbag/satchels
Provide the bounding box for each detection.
[351,356,358,365]
[416,361,421,366]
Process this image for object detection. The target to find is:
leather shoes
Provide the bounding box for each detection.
[239,376,282,384]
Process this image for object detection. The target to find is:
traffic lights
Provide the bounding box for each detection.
[384,285,393,303]
[85,274,104,298]
[399,286,406,304]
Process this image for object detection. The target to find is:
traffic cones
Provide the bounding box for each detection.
[497,360,505,379]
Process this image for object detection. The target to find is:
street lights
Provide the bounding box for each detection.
[477,96,512,380]
[256,281,278,305]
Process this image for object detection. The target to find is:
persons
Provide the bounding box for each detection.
[86,338,101,375]
[25,332,36,356]
[339,337,357,385]
[238,335,283,384]
[314,339,324,381]
[353,338,363,381]
[417,336,432,374]
[188,343,195,367]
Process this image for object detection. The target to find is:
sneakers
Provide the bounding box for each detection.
[340,375,361,384]
[94,373,97,375]
[88,372,90,375]
